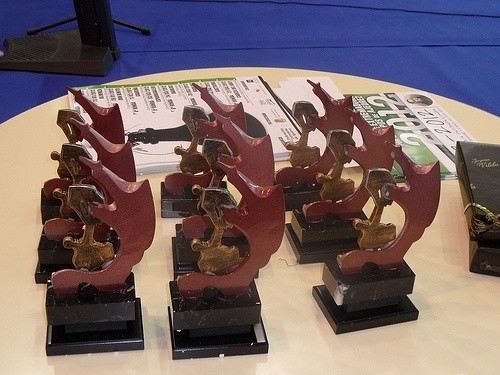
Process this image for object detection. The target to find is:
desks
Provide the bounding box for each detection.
[0,67,500,375]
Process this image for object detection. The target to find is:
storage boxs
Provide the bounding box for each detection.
[454,141,500,278]
